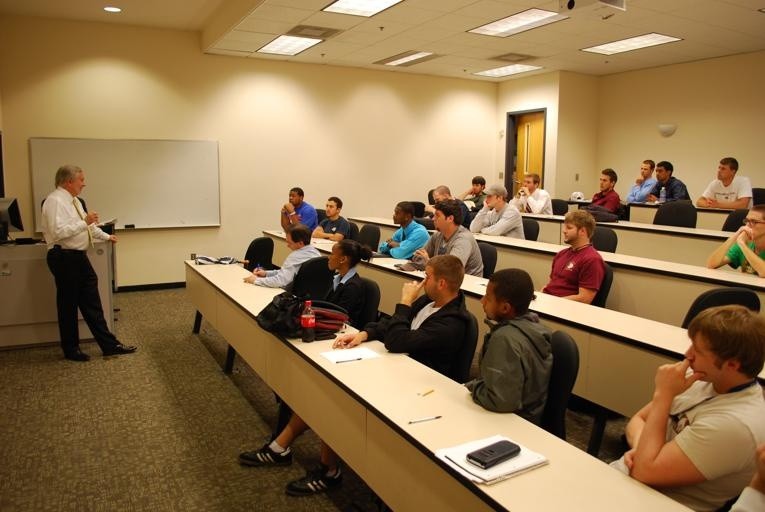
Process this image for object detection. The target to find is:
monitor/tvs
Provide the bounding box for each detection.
[0,197,24,244]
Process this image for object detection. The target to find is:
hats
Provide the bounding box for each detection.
[569,192,584,201]
[481,184,508,196]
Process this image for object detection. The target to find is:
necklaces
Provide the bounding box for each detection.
[575,243,592,250]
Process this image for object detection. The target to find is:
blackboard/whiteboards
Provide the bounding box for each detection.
[28,137,222,232]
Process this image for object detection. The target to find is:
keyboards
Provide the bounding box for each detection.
[8,238,42,243]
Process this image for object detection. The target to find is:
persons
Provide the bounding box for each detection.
[540,210,606,304]
[606,305,764,512]
[42,165,138,361]
[587,158,754,218]
[711,204,764,278]
[241,167,554,497]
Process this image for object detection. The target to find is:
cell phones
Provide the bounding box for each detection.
[466,440,521,470]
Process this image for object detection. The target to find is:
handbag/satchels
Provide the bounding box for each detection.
[256,292,348,339]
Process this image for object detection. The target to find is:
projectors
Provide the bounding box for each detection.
[558,0,626,20]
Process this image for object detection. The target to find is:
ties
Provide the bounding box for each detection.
[72,197,94,249]
[402,231,406,241]
[525,203,532,213]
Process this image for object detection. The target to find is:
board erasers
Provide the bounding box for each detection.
[124,225,134,228]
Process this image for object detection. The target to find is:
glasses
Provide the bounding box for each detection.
[744,218,765,225]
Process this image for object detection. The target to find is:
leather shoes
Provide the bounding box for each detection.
[65,349,90,361]
[103,342,136,355]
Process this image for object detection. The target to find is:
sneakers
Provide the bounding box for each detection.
[238,443,292,467]
[284,463,343,496]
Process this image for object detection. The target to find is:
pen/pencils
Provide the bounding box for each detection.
[336,358,362,363]
[422,389,434,396]
[257,263,259,270]
[409,415,443,424]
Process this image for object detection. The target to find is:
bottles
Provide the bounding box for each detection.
[659,186,667,205]
[299,299,316,342]
